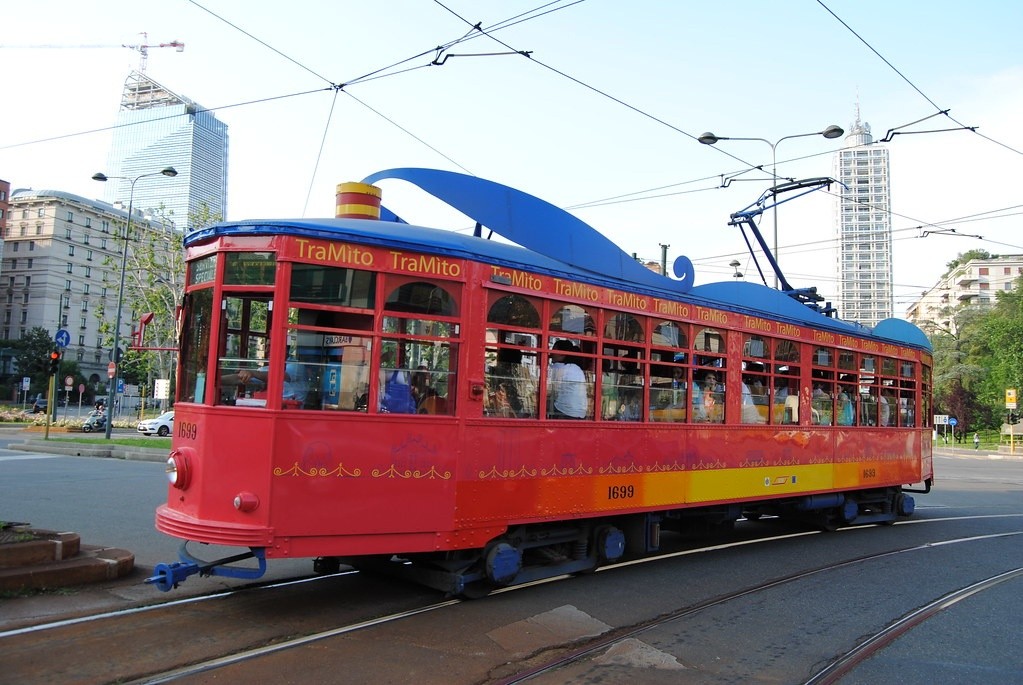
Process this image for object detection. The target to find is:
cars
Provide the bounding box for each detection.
[135,400,160,411]
[33,399,47,413]
[137,411,175,436]
[95,398,104,405]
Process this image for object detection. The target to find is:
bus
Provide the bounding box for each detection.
[145,168,936,598]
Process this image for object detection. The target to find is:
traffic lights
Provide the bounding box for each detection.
[48,349,60,374]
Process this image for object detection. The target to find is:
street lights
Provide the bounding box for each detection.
[696,125,845,292]
[91,167,179,440]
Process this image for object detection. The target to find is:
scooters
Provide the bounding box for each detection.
[82,412,114,434]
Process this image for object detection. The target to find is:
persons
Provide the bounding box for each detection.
[376,339,890,428]
[973,432,980,451]
[88,400,120,428]
[220,335,308,409]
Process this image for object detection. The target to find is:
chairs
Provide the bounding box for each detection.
[416,395,446,414]
[381,382,410,413]
[483,391,510,418]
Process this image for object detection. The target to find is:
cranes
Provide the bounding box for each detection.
[0,31,185,76]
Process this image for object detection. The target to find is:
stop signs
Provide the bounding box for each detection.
[107,362,116,378]
